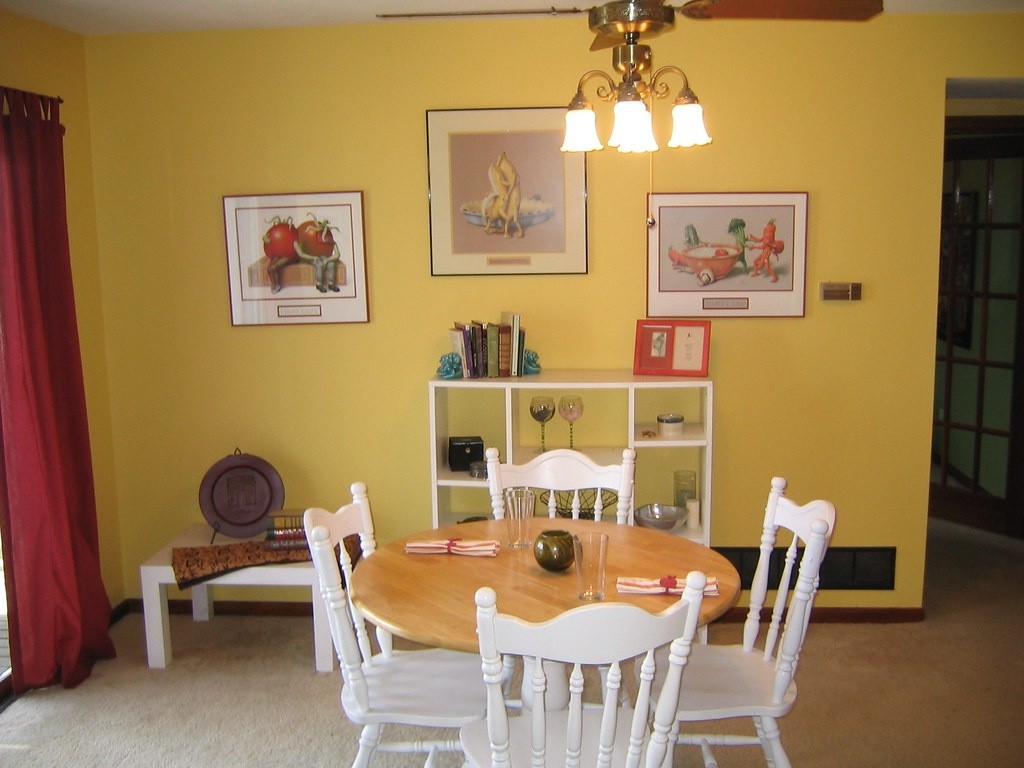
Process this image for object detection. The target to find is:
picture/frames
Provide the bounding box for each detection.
[937,190,978,349]
[222,190,371,327]
[645,193,809,319]
[425,106,588,277]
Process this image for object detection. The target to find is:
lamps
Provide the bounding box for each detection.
[559,32,713,226]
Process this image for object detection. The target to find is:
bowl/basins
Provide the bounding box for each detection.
[634,502,690,533]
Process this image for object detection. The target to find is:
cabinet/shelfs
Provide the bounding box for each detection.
[428,369,713,645]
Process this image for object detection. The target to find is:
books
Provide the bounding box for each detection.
[263,509,308,551]
[450,311,525,379]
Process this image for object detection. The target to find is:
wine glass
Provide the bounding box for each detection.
[530,395,555,454]
[558,395,584,453]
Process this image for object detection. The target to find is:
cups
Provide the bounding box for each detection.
[572,531,608,601]
[674,471,697,513]
[503,490,535,551]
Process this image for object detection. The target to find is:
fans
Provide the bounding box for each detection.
[376,0,884,152]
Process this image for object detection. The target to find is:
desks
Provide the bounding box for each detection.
[139,525,340,674]
[349,518,742,716]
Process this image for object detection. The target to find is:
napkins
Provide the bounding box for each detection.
[616,576,720,595]
[406,537,500,556]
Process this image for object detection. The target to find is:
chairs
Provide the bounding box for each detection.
[485,447,636,711]
[304,483,487,768]
[460,571,707,768]
[634,477,836,768]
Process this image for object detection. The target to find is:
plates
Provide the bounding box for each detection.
[199,454,285,538]
